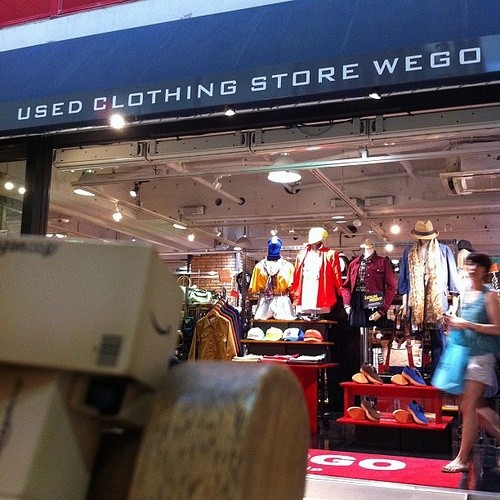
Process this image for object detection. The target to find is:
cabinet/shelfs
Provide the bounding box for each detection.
[239,298,339,344]
[337,381,454,430]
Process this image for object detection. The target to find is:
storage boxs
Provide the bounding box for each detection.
[0,235,183,500]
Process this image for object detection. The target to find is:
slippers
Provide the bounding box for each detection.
[442,463,468,472]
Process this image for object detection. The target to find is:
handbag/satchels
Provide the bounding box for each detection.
[189,288,212,305]
[176,275,197,300]
[382,312,423,368]
[431,345,470,395]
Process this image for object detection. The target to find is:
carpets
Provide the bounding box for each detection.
[304,449,464,488]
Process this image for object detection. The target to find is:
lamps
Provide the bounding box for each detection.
[112,112,401,257]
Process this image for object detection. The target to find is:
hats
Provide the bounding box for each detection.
[246,327,265,341]
[457,240,477,251]
[307,227,327,244]
[265,327,282,341]
[282,327,303,341]
[303,329,323,342]
[410,219,439,241]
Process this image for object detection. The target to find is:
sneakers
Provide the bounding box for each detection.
[361,396,380,421]
[390,373,409,385]
[407,400,428,424]
[402,365,427,387]
[392,409,415,423]
[352,372,370,384]
[347,406,379,419]
[360,361,384,384]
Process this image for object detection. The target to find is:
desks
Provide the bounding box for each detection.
[255,362,337,448]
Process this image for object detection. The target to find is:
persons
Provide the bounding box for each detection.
[248,219,460,321]
[440,252,500,473]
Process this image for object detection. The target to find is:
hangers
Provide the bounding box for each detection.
[208,293,227,318]
